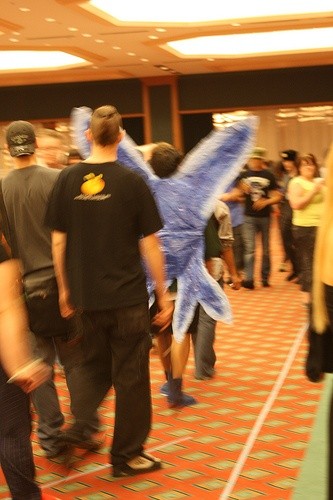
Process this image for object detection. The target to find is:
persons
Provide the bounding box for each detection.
[0,232,53,500]
[0,106,172,477]
[305,146,333,383]
[218,147,326,290]
[149,143,243,405]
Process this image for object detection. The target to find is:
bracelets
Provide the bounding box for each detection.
[5,357,46,382]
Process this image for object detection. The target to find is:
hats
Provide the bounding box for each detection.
[250,147,266,159]
[280,150,297,161]
[6,121,36,156]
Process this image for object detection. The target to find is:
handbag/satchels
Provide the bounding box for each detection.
[22,264,71,337]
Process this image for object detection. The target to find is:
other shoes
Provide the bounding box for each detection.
[48,447,75,465]
[279,262,291,271]
[262,283,271,287]
[287,272,310,291]
[168,392,195,408]
[160,382,169,395]
[67,422,100,451]
[112,453,161,477]
[241,280,254,289]
[74,415,105,430]
